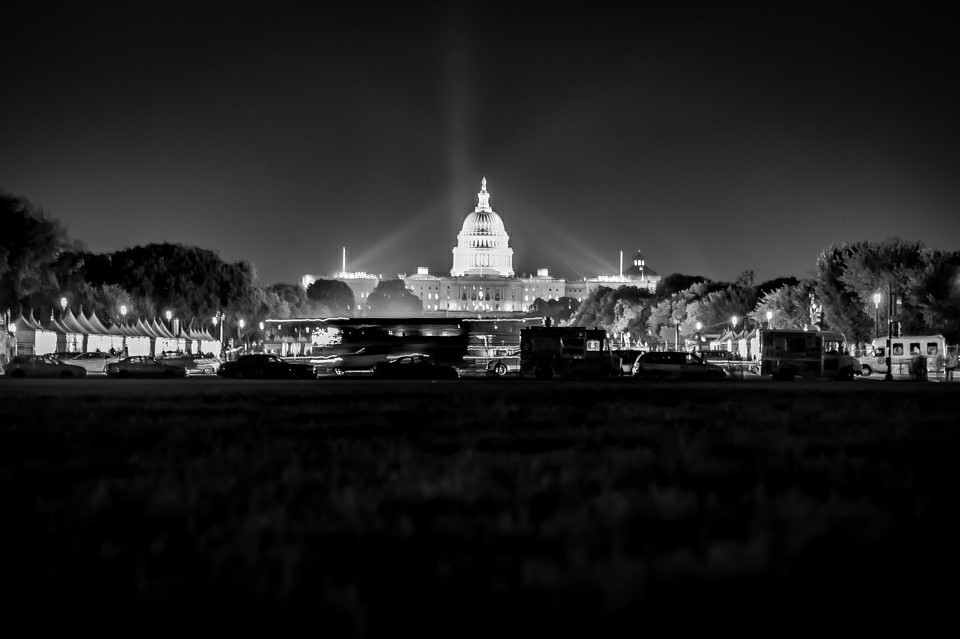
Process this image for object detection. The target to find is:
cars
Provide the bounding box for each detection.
[157,351,221,374]
[63,352,122,377]
[613,348,753,380]
[217,354,298,379]
[105,355,188,380]
[42,352,82,363]
[5,354,87,379]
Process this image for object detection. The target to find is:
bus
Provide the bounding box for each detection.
[257,317,548,377]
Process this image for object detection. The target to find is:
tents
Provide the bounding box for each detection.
[710,326,762,353]
[0,304,217,353]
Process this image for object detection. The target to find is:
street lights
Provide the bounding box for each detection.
[873,292,881,338]
[696,322,704,351]
[766,311,773,330]
[731,316,738,360]
[60,297,245,344]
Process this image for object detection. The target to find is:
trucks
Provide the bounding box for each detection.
[759,329,862,382]
[856,335,947,376]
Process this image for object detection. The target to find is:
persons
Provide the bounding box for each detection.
[935,350,957,382]
[95,346,214,359]
